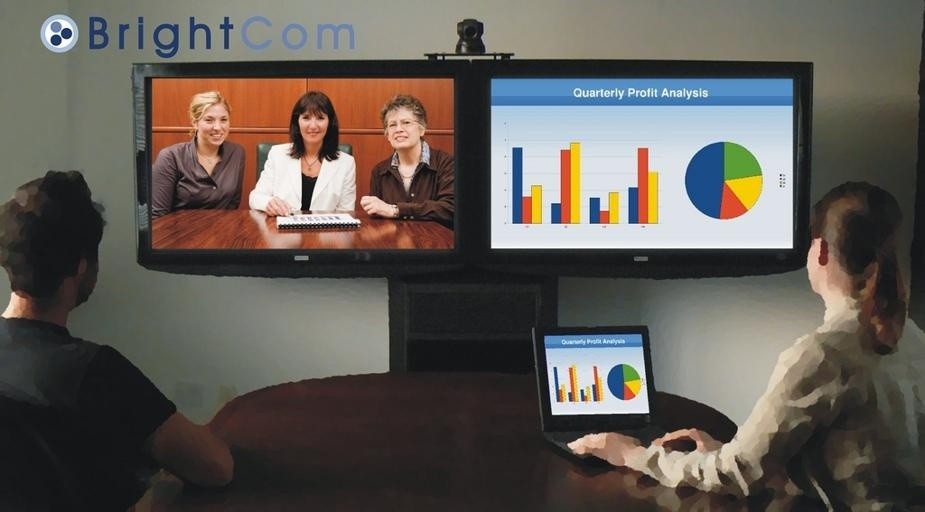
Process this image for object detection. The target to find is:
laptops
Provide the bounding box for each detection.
[530,319,682,471]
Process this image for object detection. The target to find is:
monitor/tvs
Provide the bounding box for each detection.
[129,59,473,280]
[473,57,814,278]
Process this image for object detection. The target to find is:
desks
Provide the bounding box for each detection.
[151,208,454,250]
[209,370,739,510]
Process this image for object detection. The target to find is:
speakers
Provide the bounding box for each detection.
[387,278,559,373]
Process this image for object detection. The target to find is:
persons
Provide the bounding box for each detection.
[0,171,234,511]
[567,179,925,511]
[150,90,247,226]
[361,93,453,224]
[248,91,357,217]
